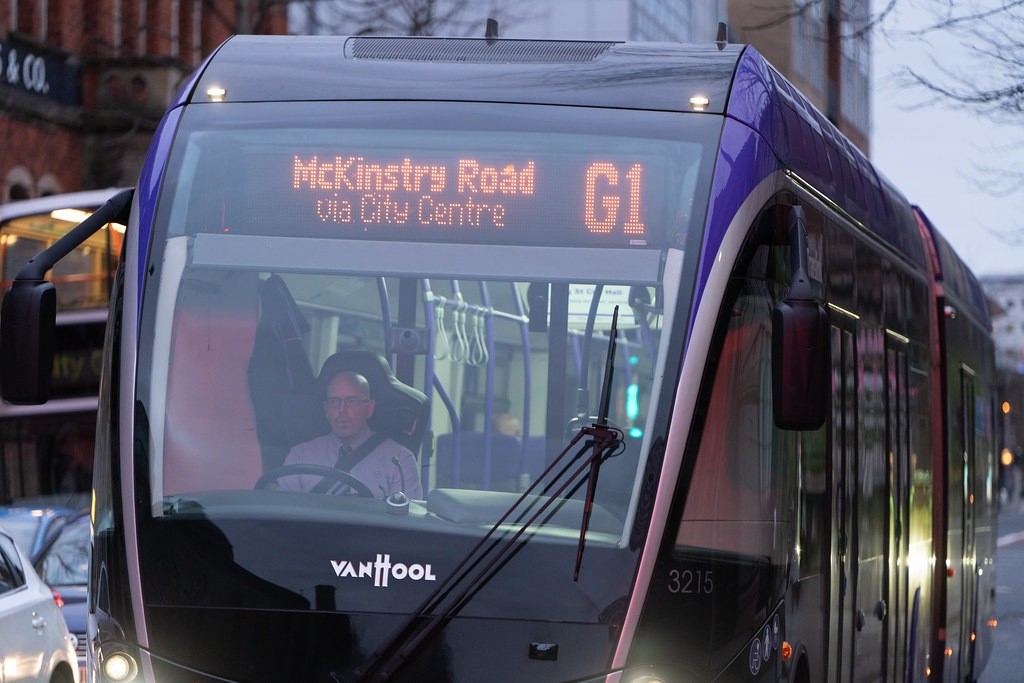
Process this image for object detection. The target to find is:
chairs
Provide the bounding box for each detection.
[525,438,544,494]
[286,351,431,461]
[436,434,522,494]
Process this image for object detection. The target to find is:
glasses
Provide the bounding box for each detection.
[321,397,369,409]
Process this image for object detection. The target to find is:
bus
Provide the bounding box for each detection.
[0,16,1012,683]
[0,183,547,671]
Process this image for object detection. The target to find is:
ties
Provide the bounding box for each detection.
[332,444,351,495]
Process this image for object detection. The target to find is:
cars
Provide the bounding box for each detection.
[0,530,84,683]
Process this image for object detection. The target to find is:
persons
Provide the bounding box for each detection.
[275,371,423,500]
[493,413,520,436]
